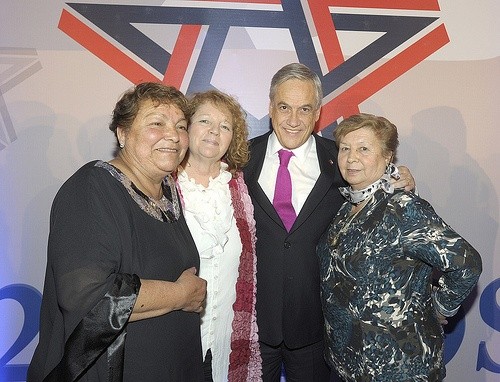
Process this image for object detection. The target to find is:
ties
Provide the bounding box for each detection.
[274,148,297,232]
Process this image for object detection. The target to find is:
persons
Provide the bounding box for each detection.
[26,81,215,382]
[174,90,264,382]
[315,113,483,382]
[226,61,415,382]
